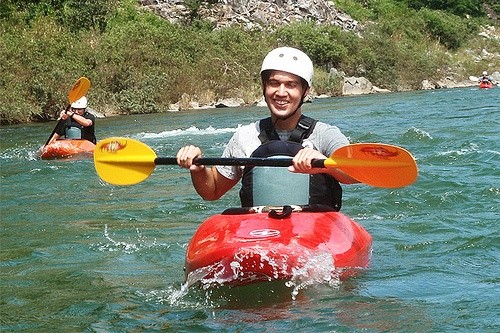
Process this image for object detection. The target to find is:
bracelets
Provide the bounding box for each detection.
[70,112,74,118]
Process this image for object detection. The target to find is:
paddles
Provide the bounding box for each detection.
[45,78,91,146]
[94,137,417,188]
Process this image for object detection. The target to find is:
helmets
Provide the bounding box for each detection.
[259,47,313,88]
[483,70,487,74]
[70,96,87,108]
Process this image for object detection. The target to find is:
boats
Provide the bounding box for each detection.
[40,139,97,161]
[480,81,492,88]
[184,205,373,287]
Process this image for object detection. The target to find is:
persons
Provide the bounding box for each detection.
[49,96,97,145]
[177,46,351,212]
